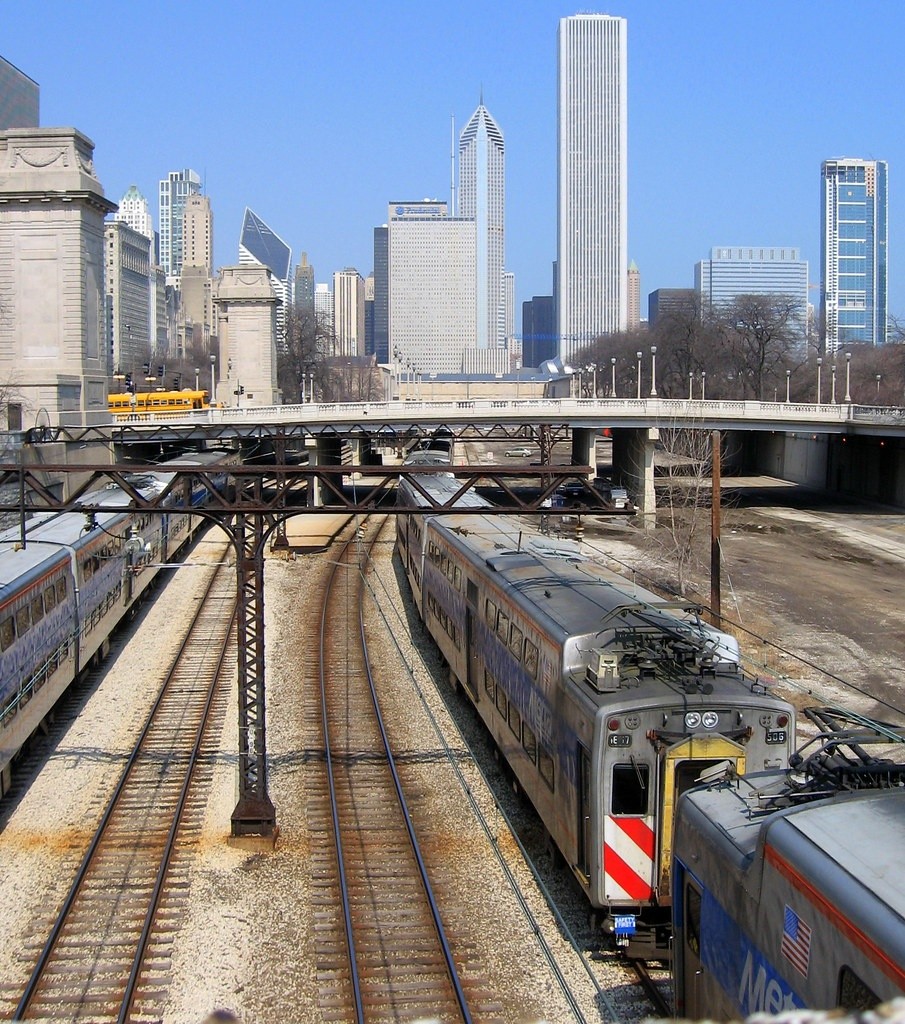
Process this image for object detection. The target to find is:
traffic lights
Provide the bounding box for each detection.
[125,372,132,386]
[174,377,179,390]
[158,365,165,377]
[144,362,151,375]
[240,385,245,394]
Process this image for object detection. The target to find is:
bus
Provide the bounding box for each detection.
[105,389,212,421]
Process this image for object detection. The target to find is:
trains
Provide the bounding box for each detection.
[0,444,246,801]
[393,429,905,1024]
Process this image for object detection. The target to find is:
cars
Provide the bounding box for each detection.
[564,482,584,499]
[503,447,532,458]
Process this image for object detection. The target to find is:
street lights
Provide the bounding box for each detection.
[688,371,694,400]
[194,367,200,392]
[701,372,706,399]
[816,358,823,405]
[591,363,598,398]
[144,377,157,392]
[637,352,643,399]
[876,373,882,392]
[831,365,836,405]
[578,367,582,400]
[786,370,791,403]
[301,374,306,404]
[650,346,659,399]
[209,355,217,405]
[393,346,400,401]
[572,368,577,399]
[397,352,423,402]
[309,373,314,403]
[611,358,617,398]
[845,353,852,402]
[774,387,778,402]
